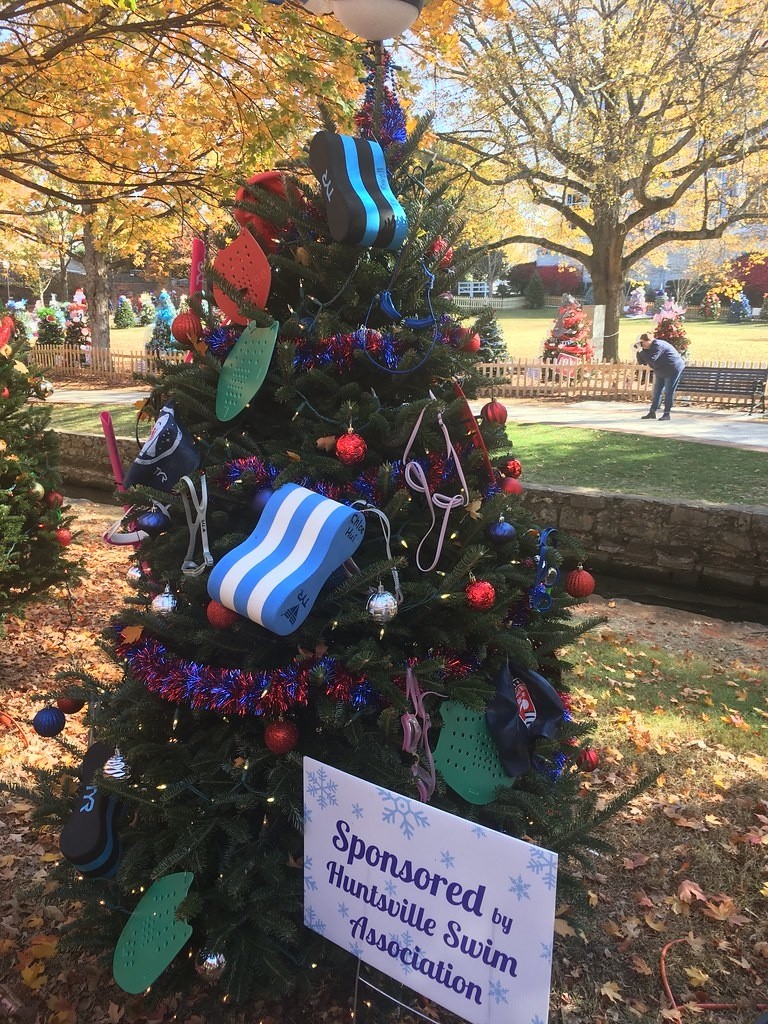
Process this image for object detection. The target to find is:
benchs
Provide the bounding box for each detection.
[676,367,768,416]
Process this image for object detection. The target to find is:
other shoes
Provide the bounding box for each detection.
[659,414,670,420]
[641,412,656,419]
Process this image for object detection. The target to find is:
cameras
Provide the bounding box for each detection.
[634,342,642,349]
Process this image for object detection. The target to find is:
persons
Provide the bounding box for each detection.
[631,333,686,421]
